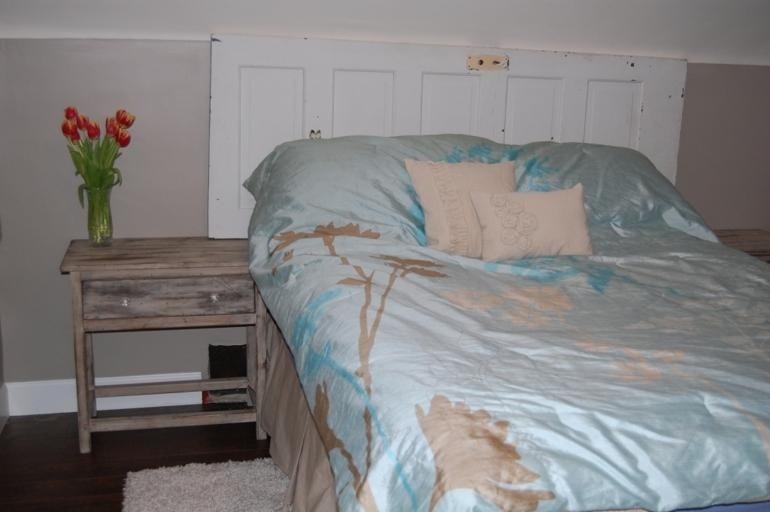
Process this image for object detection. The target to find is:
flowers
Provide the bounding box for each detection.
[56,105,135,232]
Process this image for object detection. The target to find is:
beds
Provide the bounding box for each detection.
[242,134,769,511]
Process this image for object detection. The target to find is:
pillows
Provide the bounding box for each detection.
[403,158,509,257]
[468,183,591,263]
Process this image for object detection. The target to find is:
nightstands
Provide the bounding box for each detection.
[57,235,269,453]
[710,228,769,259]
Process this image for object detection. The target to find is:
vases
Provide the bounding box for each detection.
[83,185,116,244]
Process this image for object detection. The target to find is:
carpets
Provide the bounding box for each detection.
[123,455,290,512]
